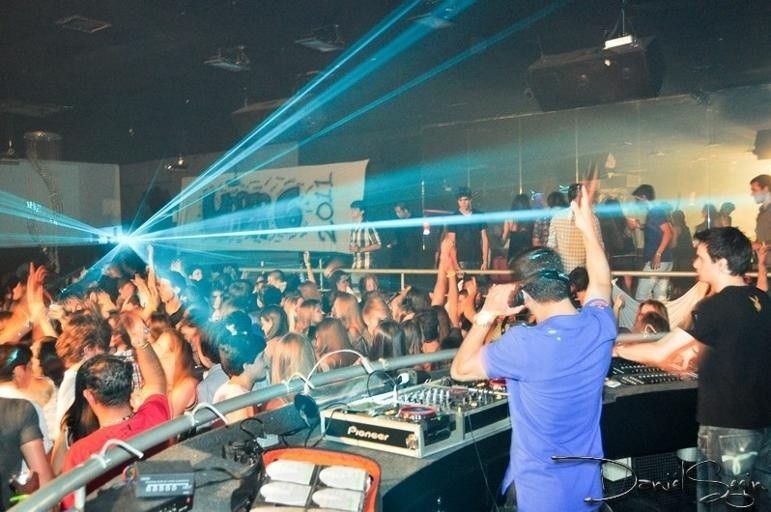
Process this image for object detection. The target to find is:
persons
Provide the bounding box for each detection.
[0,175,770,511]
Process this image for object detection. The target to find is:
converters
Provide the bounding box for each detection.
[131,456,198,500]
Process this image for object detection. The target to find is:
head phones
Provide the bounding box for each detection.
[506,269,575,307]
